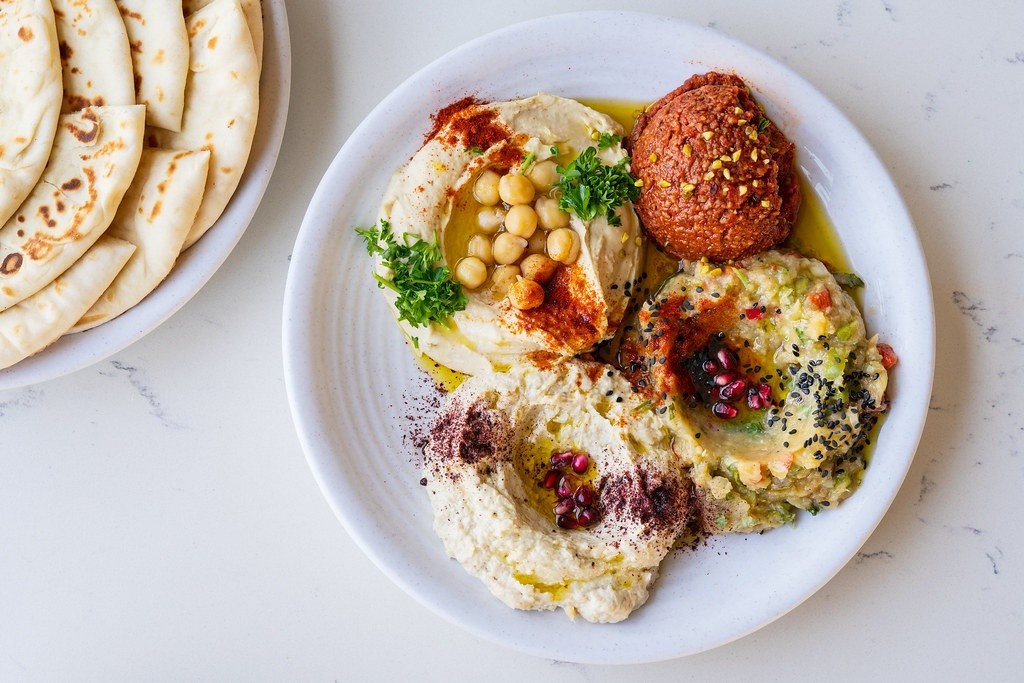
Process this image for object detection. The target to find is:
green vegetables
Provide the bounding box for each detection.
[350,130,644,353]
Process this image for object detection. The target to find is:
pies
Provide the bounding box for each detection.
[0,0,264,368]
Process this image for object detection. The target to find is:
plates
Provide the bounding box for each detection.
[279,14,935,664]
[2,1,291,396]
